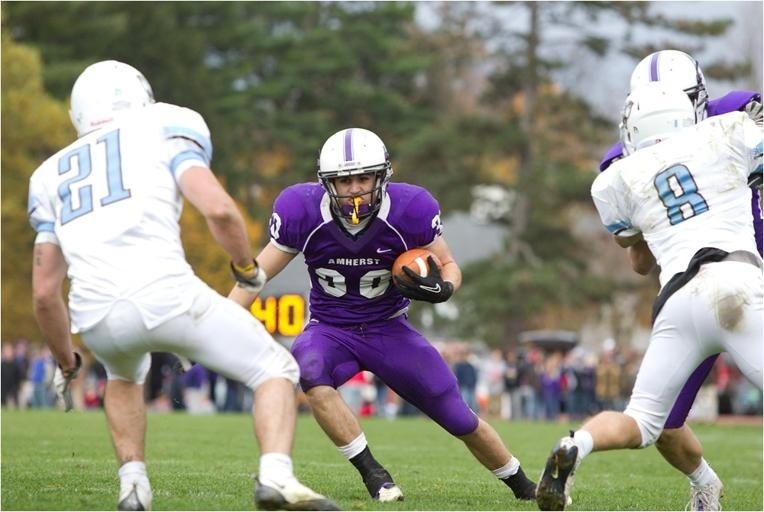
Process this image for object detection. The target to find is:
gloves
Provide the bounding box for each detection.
[54,354,80,413]
[231,261,265,293]
[393,257,453,303]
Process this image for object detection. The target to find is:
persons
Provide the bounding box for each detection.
[600,49,763,511]
[534,82,762,511]
[2,339,762,421]
[225,128,571,504]
[27,60,337,510]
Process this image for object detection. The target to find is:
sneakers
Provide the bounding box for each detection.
[253,471,340,510]
[685,476,723,511]
[366,470,402,501]
[514,481,574,505]
[536,435,582,510]
[119,484,153,511]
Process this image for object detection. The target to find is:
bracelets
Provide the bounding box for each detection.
[233,262,256,275]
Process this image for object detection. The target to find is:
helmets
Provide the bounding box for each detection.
[621,84,696,152]
[627,50,707,120]
[69,61,153,135]
[318,128,391,225]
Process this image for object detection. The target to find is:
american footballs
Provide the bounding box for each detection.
[392,248,444,290]
